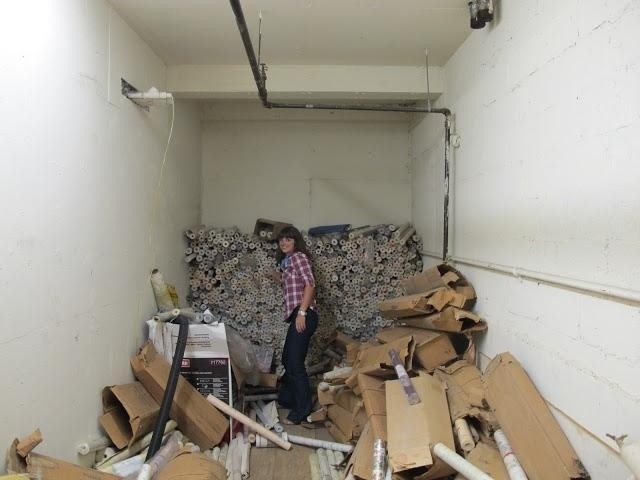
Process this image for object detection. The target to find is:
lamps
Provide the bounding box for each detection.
[468,1,495,29]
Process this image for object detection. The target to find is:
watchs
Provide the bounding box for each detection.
[297,310,307,317]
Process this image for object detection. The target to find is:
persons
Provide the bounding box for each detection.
[264,226,319,426]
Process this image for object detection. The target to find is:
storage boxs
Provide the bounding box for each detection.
[147,320,240,420]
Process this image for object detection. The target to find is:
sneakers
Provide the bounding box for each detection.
[282,415,308,425]
